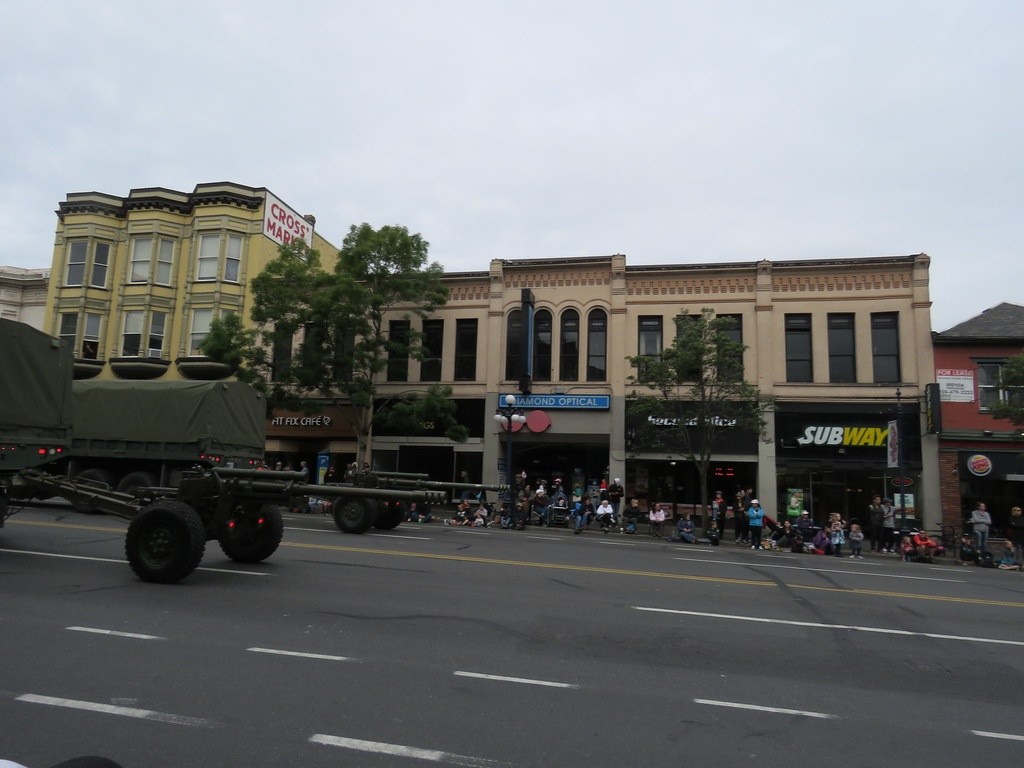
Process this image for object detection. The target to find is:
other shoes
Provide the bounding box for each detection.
[738,537,741,541]
[857,555,864,559]
[736,539,740,542]
[742,540,748,543]
[849,555,855,559]
[751,545,755,549]
[963,561,967,566]
[888,548,895,553]
[759,546,763,550]
[882,547,887,552]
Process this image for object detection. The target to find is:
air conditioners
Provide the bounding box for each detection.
[147,348,162,359]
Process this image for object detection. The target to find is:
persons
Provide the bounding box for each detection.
[814,526,835,554]
[291,461,371,514]
[256,461,294,470]
[447,489,488,527]
[960,502,991,560]
[732,488,813,548]
[510,473,569,530]
[408,505,432,523]
[830,513,846,557]
[707,491,727,545]
[868,496,901,552]
[622,499,640,535]
[649,503,665,537]
[677,512,697,544]
[499,505,511,528]
[849,524,864,559]
[572,478,624,534]
[998,507,1024,570]
[900,530,936,562]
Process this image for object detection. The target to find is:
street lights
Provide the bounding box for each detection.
[493,394,527,509]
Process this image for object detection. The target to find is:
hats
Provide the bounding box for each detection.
[750,499,759,504]
[714,491,723,496]
[555,478,561,483]
[802,510,809,515]
[883,497,893,503]
